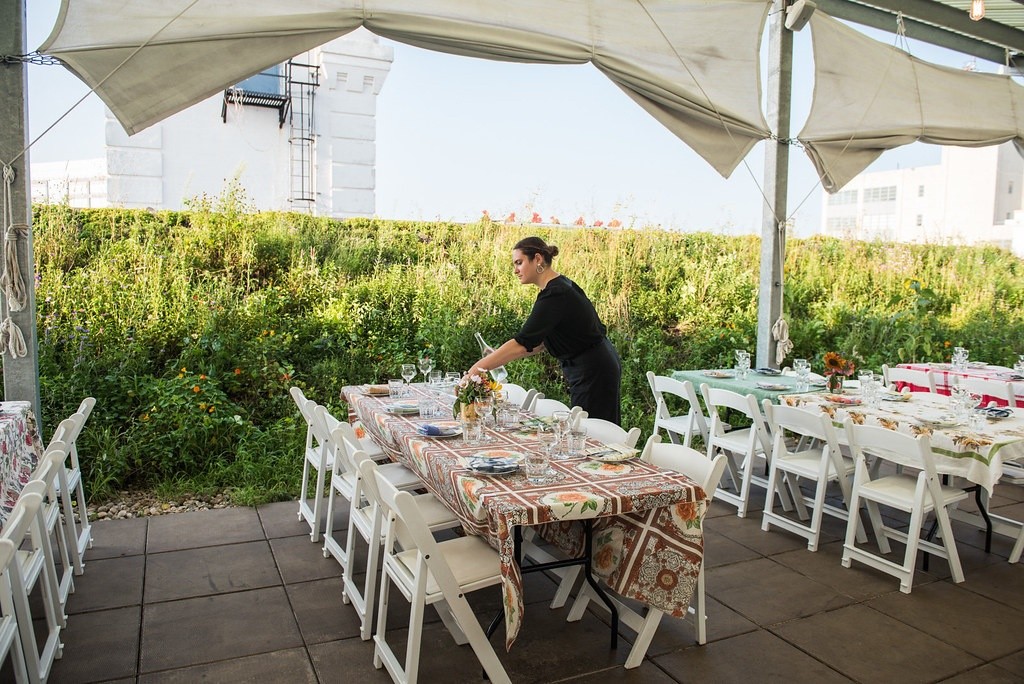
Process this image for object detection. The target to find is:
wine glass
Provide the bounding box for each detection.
[734,349,750,380]
[551,411,571,460]
[401,358,433,398]
[953,347,969,373]
[537,422,561,468]
[856,368,885,409]
[952,384,983,434]
[473,395,494,443]
[494,390,508,432]
[792,359,811,393]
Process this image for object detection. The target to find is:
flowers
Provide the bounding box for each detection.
[823,351,855,393]
[453,367,502,422]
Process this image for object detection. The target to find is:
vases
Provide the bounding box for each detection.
[826,375,843,393]
[460,402,476,420]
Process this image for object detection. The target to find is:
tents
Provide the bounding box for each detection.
[1,0,1024,436]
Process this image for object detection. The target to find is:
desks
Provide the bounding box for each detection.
[674,364,1024,573]
[0,401,46,533]
[338,383,712,680]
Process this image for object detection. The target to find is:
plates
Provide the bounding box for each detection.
[828,399,859,406]
[588,454,636,463]
[462,463,517,475]
[703,371,735,378]
[361,391,387,397]
[415,426,463,438]
[757,383,792,391]
[386,411,419,415]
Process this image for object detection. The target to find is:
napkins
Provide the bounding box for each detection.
[585,442,641,461]
[465,456,520,473]
[702,370,734,377]
[757,367,782,375]
[364,385,389,394]
[982,407,1013,418]
[518,417,553,428]
[417,423,463,436]
[385,405,420,414]
[757,382,791,390]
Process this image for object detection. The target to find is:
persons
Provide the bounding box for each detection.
[455,236,622,428]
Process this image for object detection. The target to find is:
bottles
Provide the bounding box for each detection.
[474,332,508,382]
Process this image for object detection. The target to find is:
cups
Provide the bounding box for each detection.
[388,371,461,420]
[1013,355,1024,377]
[525,449,550,485]
[501,403,521,429]
[968,408,987,435]
[735,364,747,381]
[462,417,481,446]
[566,425,587,458]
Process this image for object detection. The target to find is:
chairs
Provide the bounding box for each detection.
[0,397,97,684]
[289,363,1024,684]
